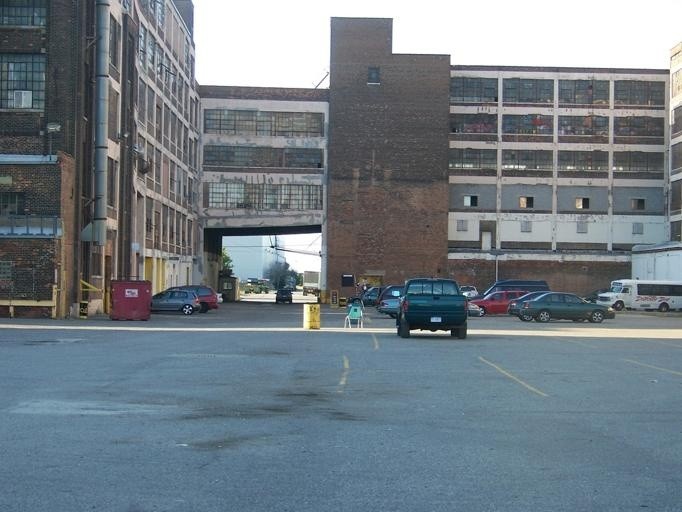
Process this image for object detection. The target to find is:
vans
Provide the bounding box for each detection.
[151,289,201,315]
[482,279,550,299]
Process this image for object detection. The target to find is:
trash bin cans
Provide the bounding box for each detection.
[303,304,320,330]
[110,280,152,321]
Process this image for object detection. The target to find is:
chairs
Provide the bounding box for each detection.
[343,306,363,328]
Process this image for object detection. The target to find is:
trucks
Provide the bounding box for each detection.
[303,270,320,297]
[244,278,269,294]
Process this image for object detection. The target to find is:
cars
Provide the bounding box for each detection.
[379,291,485,317]
[217,293,224,304]
[506,290,586,323]
[469,291,531,316]
[522,292,616,324]
[459,285,479,299]
[348,287,378,306]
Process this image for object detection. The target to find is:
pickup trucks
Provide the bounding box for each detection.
[397,277,469,339]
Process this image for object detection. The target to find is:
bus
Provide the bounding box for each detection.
[596,279,682,313]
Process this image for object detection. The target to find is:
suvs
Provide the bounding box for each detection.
[376,284,446,319]
[168,285,218,314]
[275,289,293,304]
